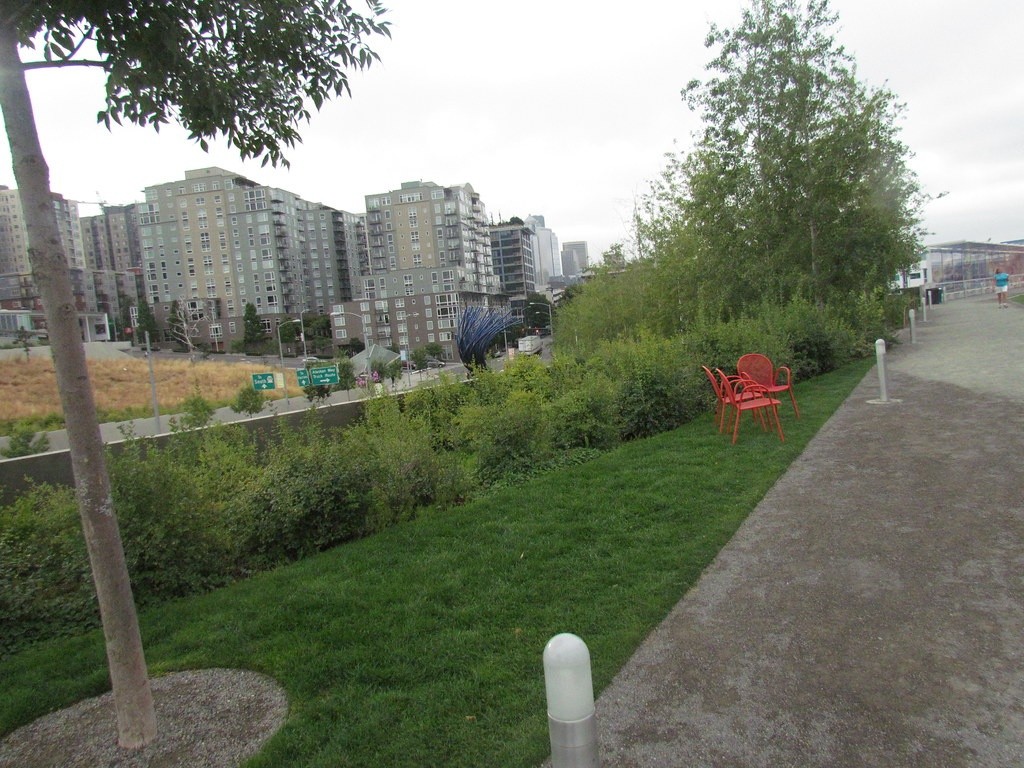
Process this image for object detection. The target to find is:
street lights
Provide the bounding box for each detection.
[402,313,419,385]
[277,319,302,404]
[530,302,553,340]
[331,312,375,398]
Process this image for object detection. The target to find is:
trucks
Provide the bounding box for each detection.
[518,336,543,357]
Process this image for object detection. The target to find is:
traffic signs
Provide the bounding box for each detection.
[252,373,276,390]
[296,369,310,387]
[309,367,339,385]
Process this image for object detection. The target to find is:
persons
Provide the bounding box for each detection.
[991,268,1009,309]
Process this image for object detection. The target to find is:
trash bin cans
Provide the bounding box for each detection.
[925,287,942,305]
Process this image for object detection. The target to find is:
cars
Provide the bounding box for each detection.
[427,358,446,368]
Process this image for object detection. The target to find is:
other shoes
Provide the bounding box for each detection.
[998,305,1001,308]
[1003,303,1008,308]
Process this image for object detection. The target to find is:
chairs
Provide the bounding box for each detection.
[736,354,800,427]
[715,367,786,444]
[701,365,772,434]
